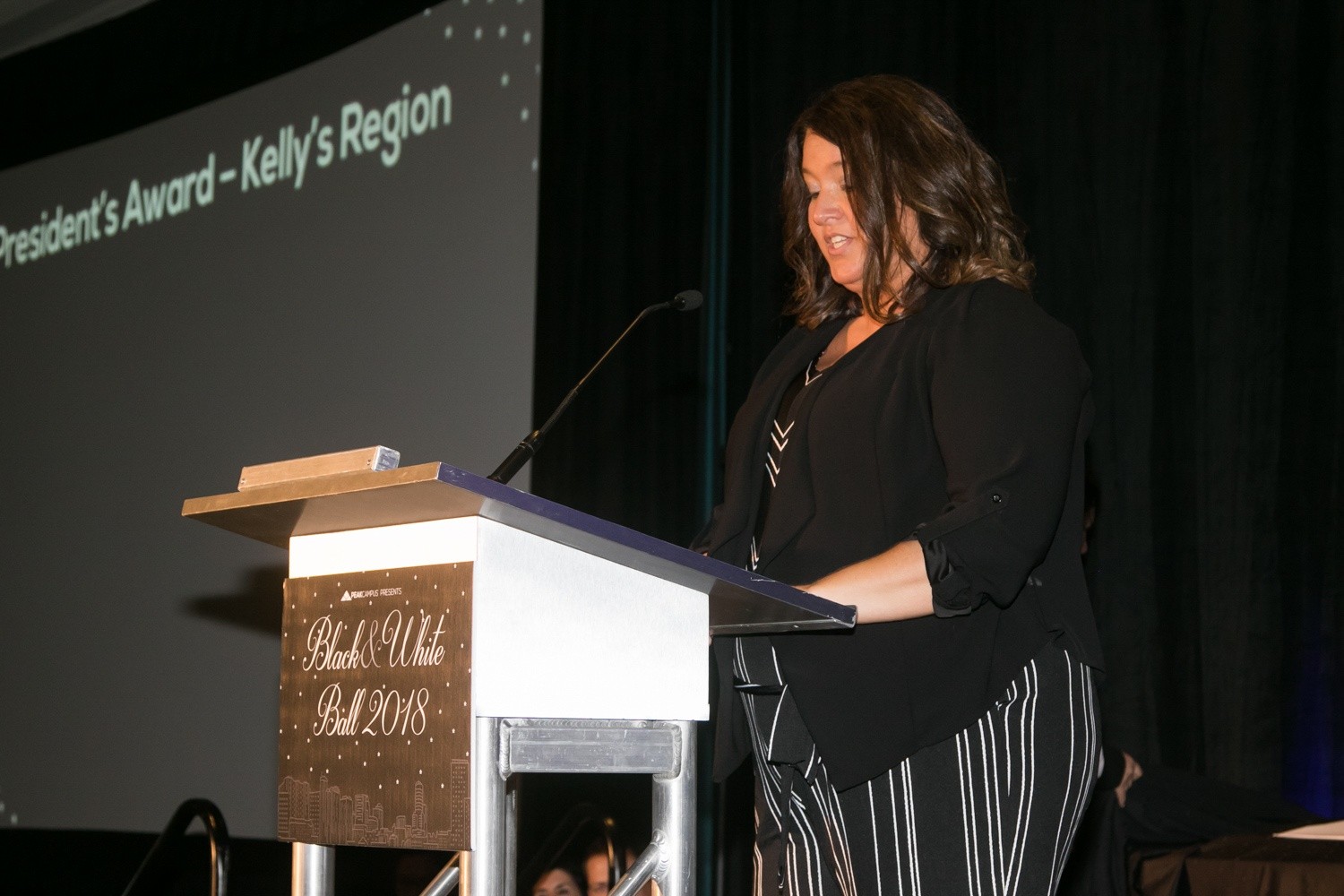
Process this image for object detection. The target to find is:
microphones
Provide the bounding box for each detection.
[488,288,704,484]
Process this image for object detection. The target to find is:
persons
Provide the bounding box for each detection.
[532,833,651,896]
[709,74,1147,896]
